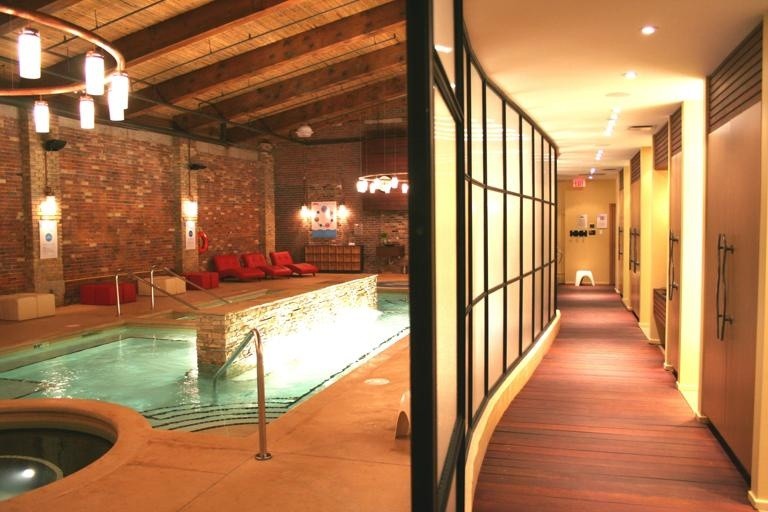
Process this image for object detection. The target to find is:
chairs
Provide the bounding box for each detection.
[214,250,318,282]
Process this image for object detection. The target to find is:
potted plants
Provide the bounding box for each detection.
[381,233,387,245]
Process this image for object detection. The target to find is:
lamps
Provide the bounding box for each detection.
[356,112,409,194]
[296,125,313,136]
[0,5,129,133]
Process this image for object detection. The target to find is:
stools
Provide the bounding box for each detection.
[575,270,595,287]
[0,271,219,322]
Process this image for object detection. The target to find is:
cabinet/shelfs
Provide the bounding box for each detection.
[305,245,364,273]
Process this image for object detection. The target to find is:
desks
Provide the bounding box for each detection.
[376,245,405,273]
